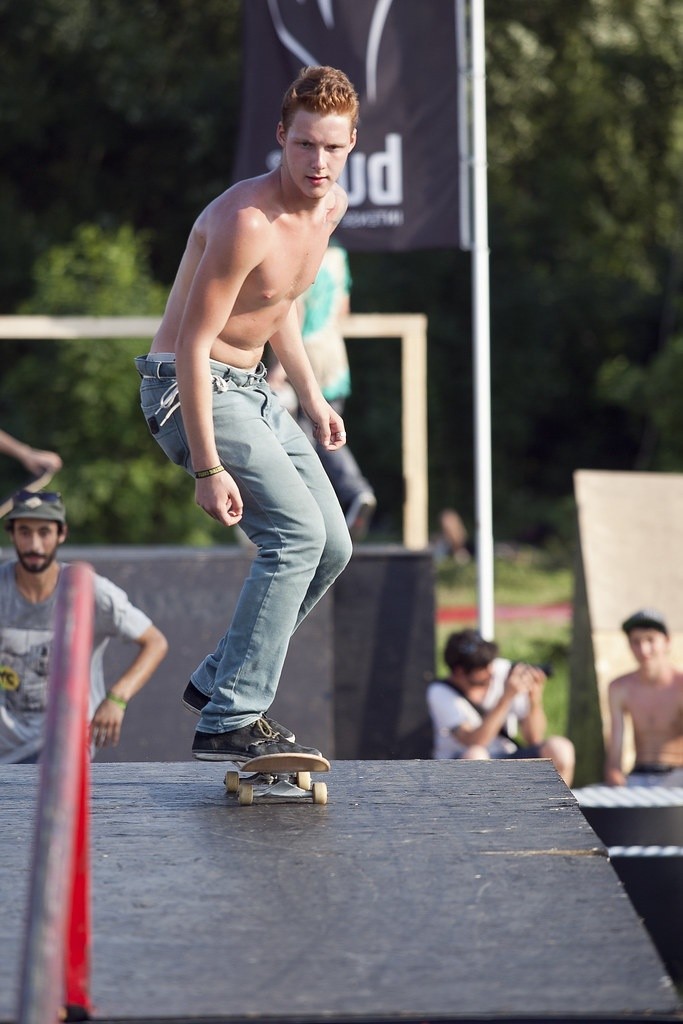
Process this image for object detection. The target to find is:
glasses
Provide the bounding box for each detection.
[14,490,64,504]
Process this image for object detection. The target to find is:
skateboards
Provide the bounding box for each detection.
[223,749,333,809]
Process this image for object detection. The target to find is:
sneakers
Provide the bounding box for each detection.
[192,718,322,763]
[181,679,295,743]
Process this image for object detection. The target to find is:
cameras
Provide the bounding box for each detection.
[511,659,553,678]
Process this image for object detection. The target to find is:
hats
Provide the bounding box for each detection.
[0,499,66,531]
[444,630,497,669]
[622,610,670,639]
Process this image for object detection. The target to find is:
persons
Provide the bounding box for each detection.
[0,489,169,762]
[604,606,683,791]
[260,233,377,546]
[426,469,475,583]
[134,65,356,763]
[425,626,575,789]
[0,428,62,478]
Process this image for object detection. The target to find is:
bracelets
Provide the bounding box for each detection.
[106,690,128,711]
[194,465,224,478]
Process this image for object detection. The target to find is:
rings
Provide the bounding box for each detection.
[336,432,346,437]
